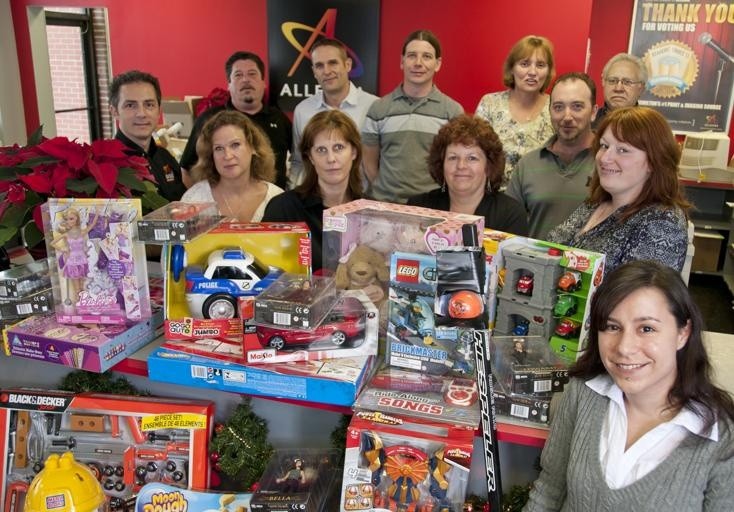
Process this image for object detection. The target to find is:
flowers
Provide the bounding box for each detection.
[0,120,174,262]
[193,87,231,116]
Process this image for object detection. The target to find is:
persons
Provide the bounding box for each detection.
[474,36,698,273]
[362,431,450,511]
[109,50,293,224]
[50,205,101,301]
[275,459,305,493]
[261,30,528,275]
[521,260,734,512]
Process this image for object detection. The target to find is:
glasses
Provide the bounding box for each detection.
[604,77,641,86]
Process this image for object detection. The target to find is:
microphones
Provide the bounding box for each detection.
[697,32,734,65]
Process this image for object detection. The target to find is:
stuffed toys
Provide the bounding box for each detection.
[334,245,391,323]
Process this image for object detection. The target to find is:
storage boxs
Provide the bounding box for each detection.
[162,95,205,140]
